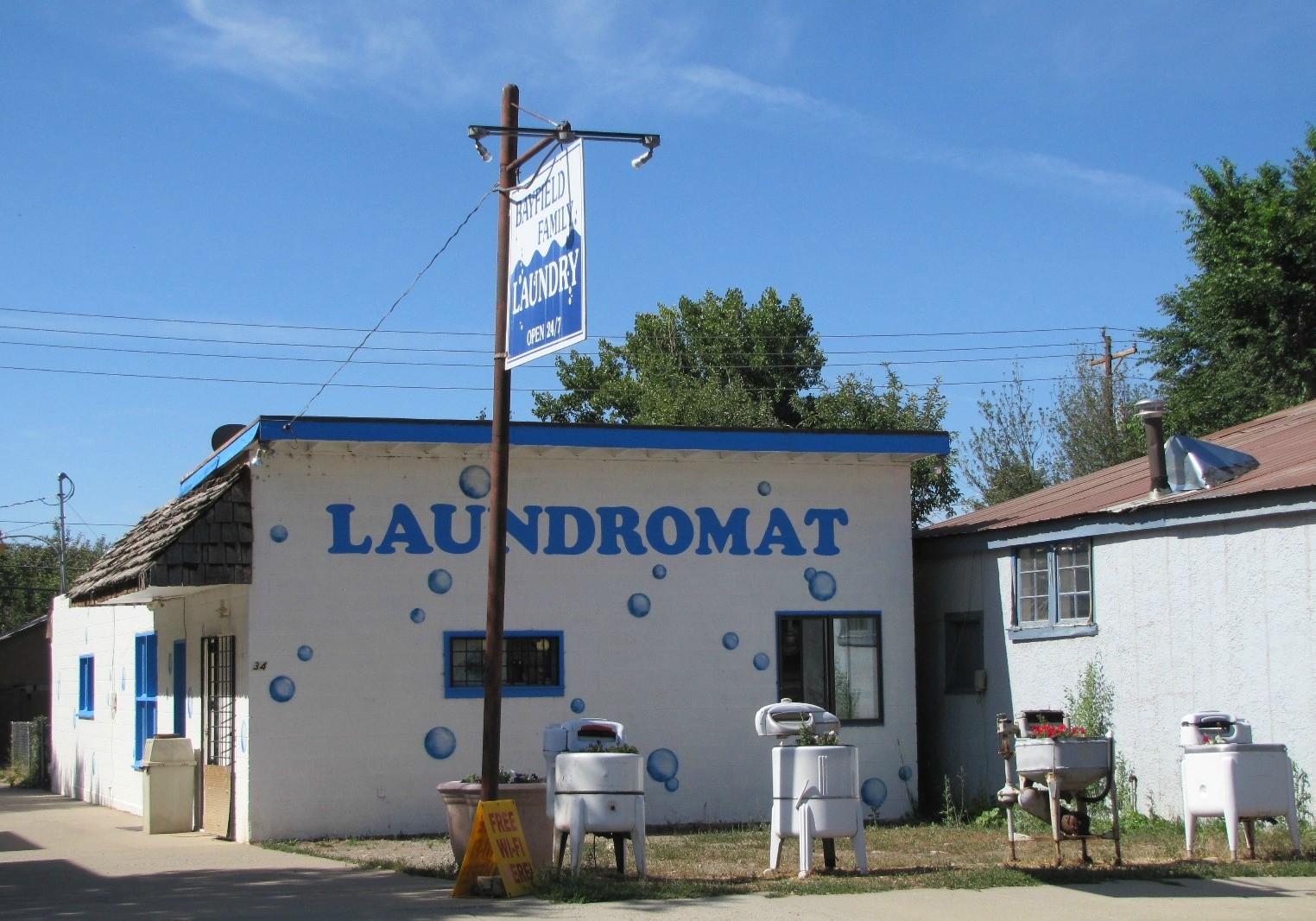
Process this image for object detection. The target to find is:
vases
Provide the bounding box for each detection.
[463,782,553,876]
[437,778,473,868]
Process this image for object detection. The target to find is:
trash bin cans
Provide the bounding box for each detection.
[139,737,197,835]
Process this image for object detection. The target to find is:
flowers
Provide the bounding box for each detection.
[1201,732,1231,744]
[461,766,545,785]
[1030,723,1089,738]
